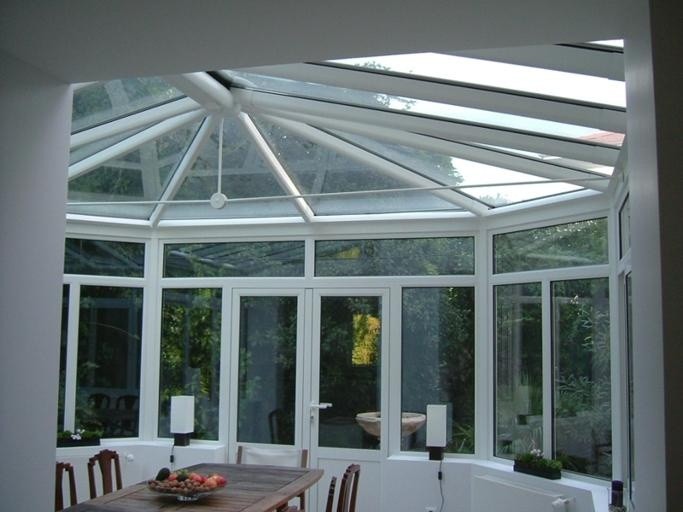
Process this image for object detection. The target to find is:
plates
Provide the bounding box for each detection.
[149,484,226,503]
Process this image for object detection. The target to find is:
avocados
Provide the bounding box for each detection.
[155,468,169,480]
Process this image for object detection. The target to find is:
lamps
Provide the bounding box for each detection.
[426,404,447,460]
[170,396,195,445]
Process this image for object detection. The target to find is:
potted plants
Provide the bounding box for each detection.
[514,452,563,480]
[57,430,104,447]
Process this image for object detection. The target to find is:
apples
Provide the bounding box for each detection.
[168,472,227,489]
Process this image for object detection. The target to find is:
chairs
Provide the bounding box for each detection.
[85,394,138,437]
[268,409,293,444]
[87,449,123,499]
[55,461,77,512]
[236,446,309,512]
[325,464,360,512]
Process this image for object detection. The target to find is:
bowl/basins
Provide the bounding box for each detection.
[356,410,425,438]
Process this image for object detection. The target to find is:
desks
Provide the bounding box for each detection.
[55,463,324,512]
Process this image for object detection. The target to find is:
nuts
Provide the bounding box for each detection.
[147,479,210,494]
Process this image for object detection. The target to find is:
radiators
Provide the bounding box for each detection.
[470,474,564,512]
[56,454,126,507]
